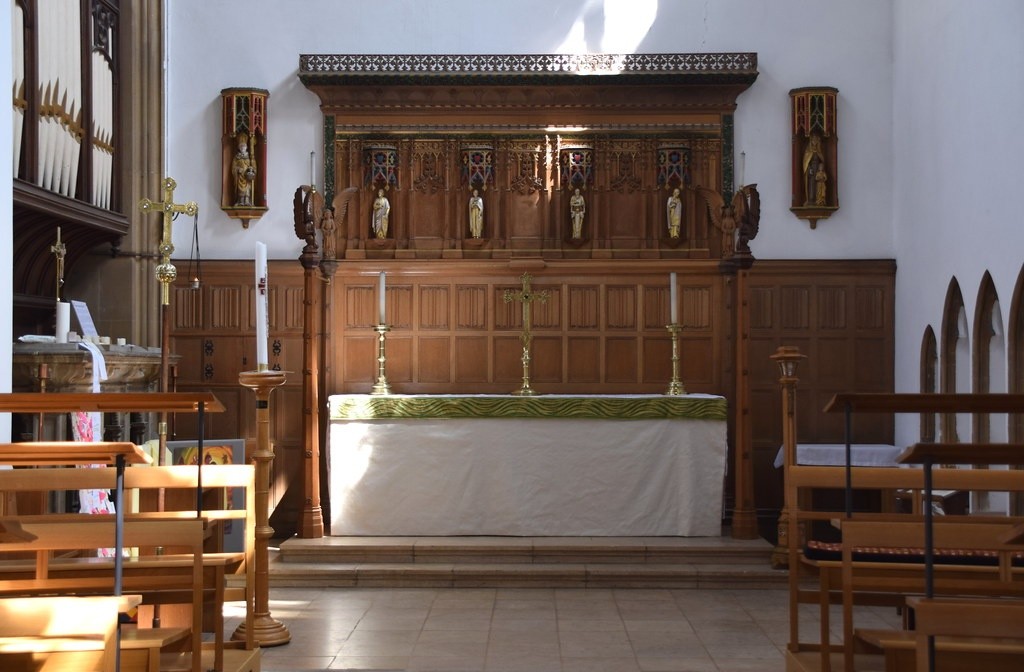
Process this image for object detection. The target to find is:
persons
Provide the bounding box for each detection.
[372,189,390,239]
[815,163,827,206]
[469,190,483,239]
[232,134,251,206]
[322,209,336,259]
[803,134,825,206]
[667,189,681,239]
[570,189,585,240]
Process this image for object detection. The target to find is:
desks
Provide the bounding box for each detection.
[325,394,728,537]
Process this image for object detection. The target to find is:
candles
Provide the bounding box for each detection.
[310,151,316,185]
[56,302,70,344]
[255,240,269,363]
[739,151,746,185]
[671,272,677,323]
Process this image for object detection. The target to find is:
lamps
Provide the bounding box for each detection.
[379,270,386,324]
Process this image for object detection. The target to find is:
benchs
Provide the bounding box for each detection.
[1,437,275,672]
[772,339,1024,672]
